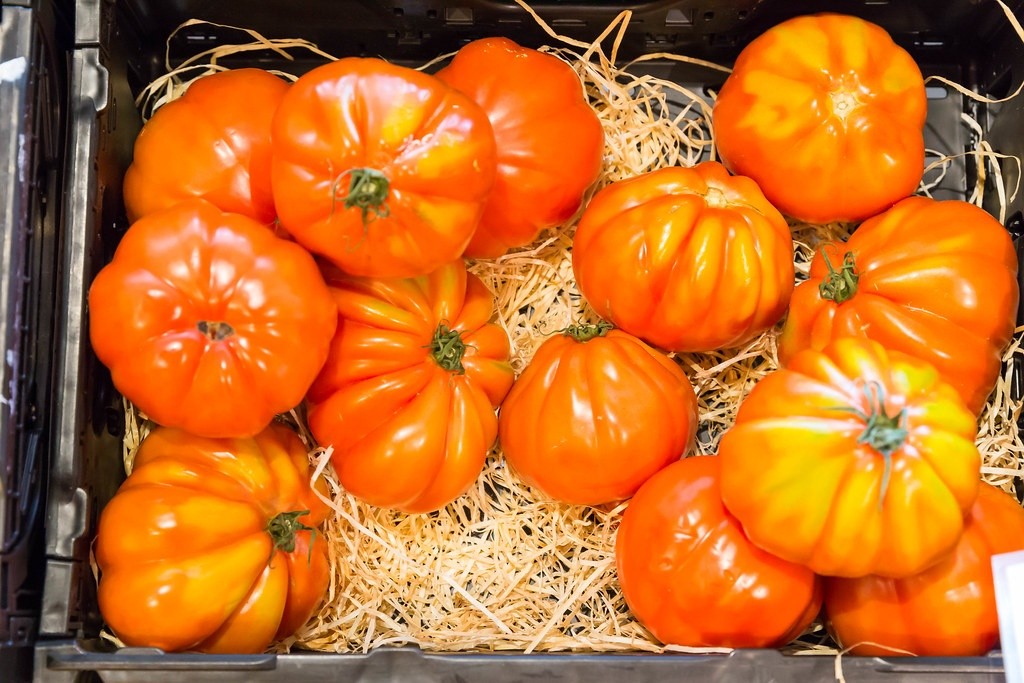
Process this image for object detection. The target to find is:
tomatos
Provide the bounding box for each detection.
[91,12,1024,658]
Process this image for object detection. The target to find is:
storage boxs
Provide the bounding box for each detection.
[33,0,1024,683]
